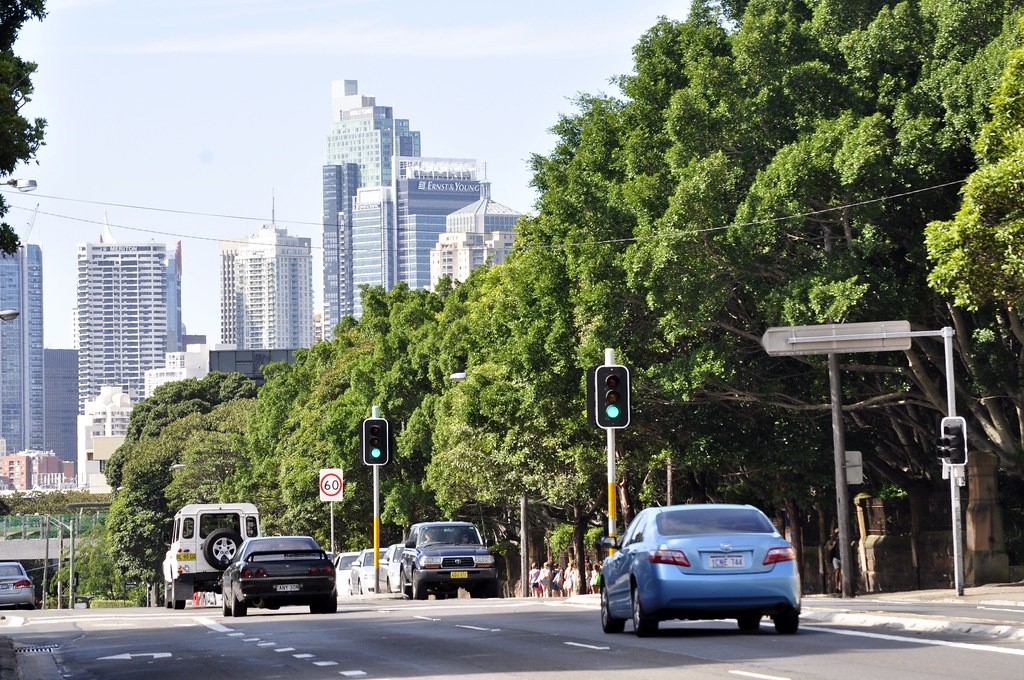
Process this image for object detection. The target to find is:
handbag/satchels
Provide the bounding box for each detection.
[595,572,601,586]
[563,575,572,590]
[552,570,563,588]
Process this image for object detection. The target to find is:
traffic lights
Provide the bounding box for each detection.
[935,419,966,465]
[594,364,631,430]
[362,418,388,466]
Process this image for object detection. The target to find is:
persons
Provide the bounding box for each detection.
[529,557,603,597]
[826,527,842,593]
[420,531,437,545]
[458,532,472,543]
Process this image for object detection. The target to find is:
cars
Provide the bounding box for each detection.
[378,543,406,593]
[333,552,362,596]
[348,548,389,595]
[218,535,339,618]
[597,503,802,635]
[0,562,36,608]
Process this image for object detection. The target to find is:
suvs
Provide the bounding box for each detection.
[399,521,497,600]
[162,503,261,610]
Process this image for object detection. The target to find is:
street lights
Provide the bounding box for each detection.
[15,512,75,609]
[447,372,530,599]
